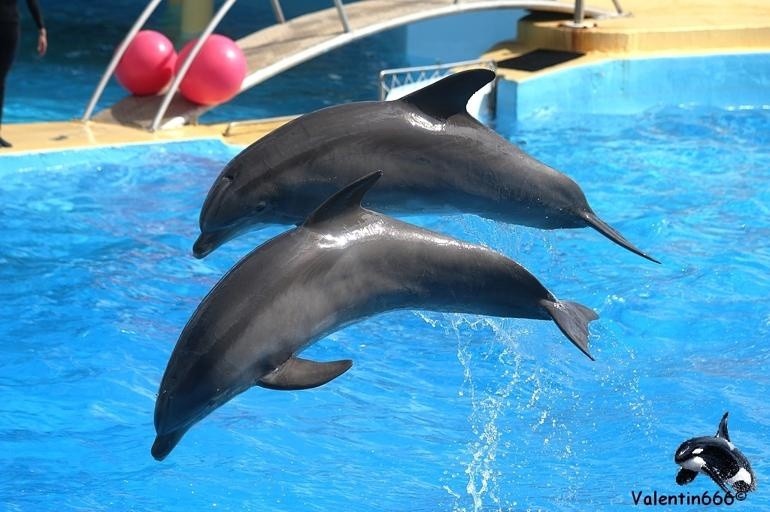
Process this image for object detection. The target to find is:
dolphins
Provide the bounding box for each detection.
[151,169,600,461]
[193,69,661,265]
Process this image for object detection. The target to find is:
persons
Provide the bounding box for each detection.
[0,1,49,149]
